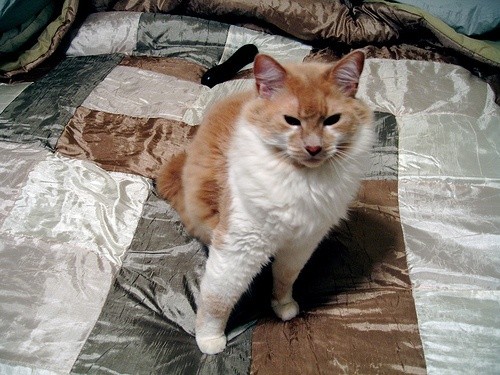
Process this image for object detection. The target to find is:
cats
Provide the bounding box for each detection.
[156,49,375,354]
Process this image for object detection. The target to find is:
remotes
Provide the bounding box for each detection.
[201,44,262,88]
